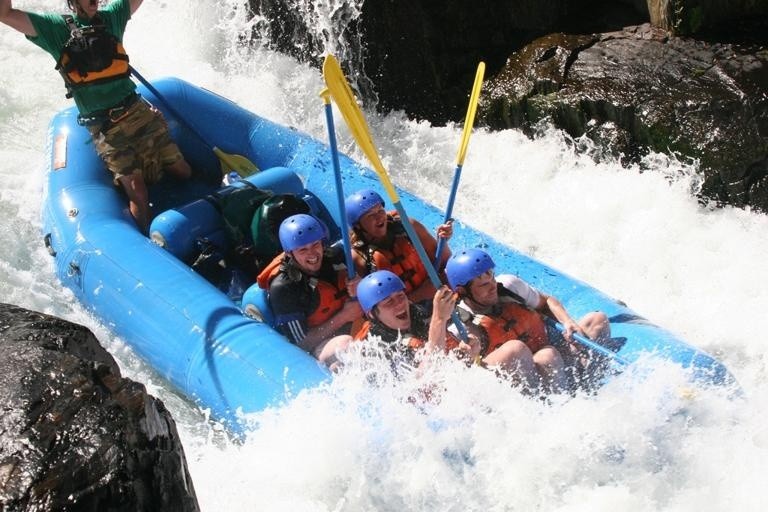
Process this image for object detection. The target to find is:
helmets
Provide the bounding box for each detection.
[446,249,495,291]
[357,270,406,314]
[279,214,326,253]
[344,189,384,230]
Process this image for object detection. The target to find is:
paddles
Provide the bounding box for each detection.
[323,54,480,364]
[129,65,261,178]
[434,61,486,271]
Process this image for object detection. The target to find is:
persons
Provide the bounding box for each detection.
[0,0,195,237]
[267,190,612,402]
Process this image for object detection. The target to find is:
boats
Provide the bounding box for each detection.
[39,66,746,493]
